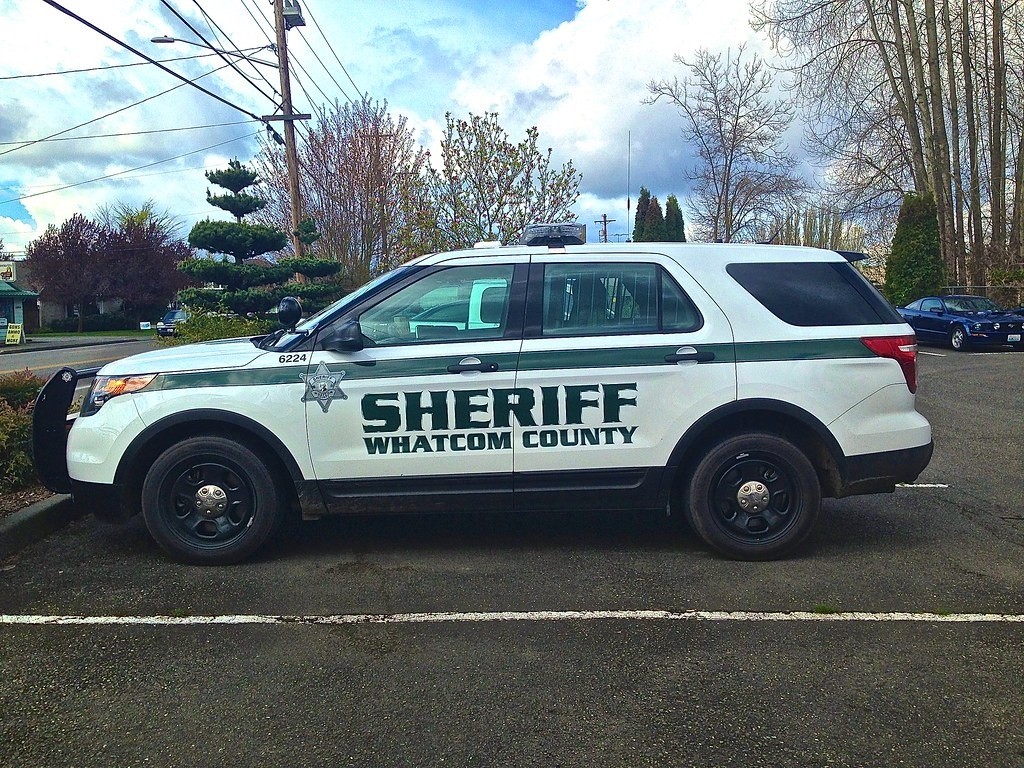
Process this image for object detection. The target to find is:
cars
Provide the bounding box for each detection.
[156,310,192,338]
[894,295,1024,352]
[30,223,934,562]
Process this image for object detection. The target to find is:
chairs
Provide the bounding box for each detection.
[567,277,612,324]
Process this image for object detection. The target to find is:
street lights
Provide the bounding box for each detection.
[150,36,310,320]
